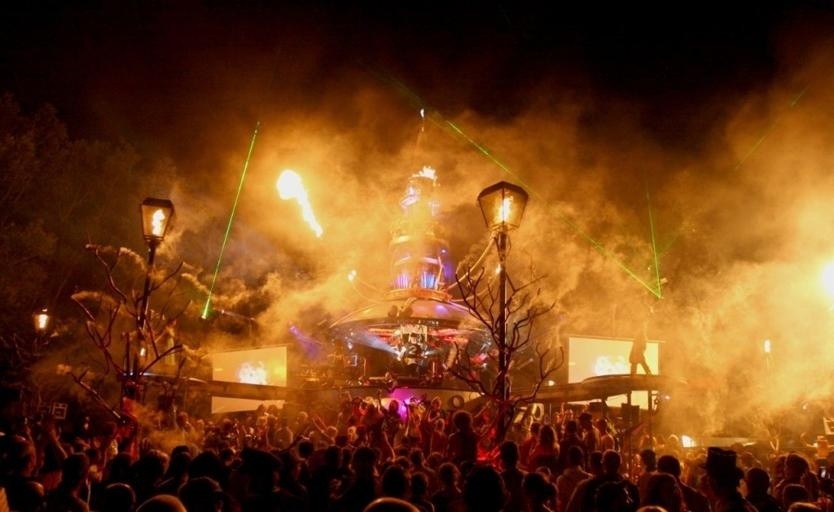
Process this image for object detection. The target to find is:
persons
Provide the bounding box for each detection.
[403,333,424,384]
[1,386,834,512]
[628,318,652,375]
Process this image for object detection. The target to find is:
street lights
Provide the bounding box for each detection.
[126,196,175,420]
[477,179,529,438]
[31,308,51,353]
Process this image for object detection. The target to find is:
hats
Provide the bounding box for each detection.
[698,446,747,475]
